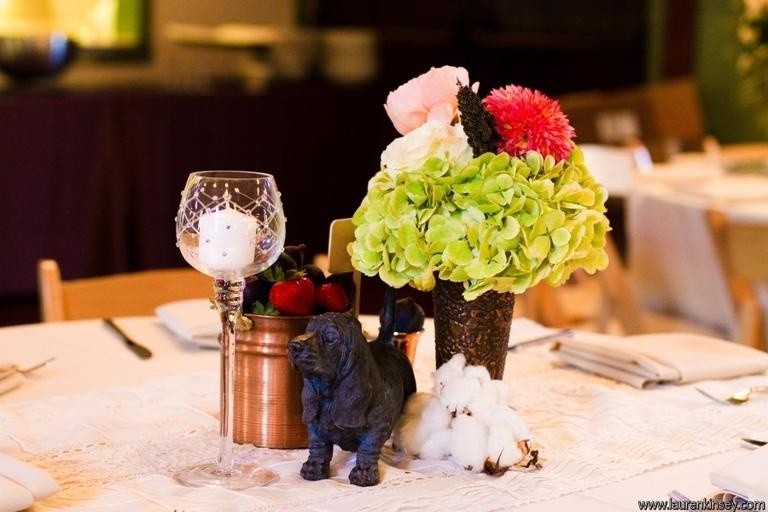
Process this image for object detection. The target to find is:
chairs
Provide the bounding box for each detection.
[36,255,223,325]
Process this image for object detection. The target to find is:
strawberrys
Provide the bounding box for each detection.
[315,283,350,313]
[264,265,315,317]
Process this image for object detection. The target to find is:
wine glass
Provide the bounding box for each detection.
[176,169,288,490]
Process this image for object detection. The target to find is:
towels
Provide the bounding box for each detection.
[155,297,225,353]
[547,330,764,390]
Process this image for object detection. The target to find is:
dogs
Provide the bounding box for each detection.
[286,284,416,487]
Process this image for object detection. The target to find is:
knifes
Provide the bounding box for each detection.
[102,316,152,361]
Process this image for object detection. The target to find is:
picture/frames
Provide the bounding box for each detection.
[0,3,157,62]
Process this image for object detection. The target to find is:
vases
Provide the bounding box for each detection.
[427,270,513,380]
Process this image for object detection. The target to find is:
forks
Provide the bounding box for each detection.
[695,383,768,406]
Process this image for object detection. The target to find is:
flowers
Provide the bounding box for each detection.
[347,62,614,304]
